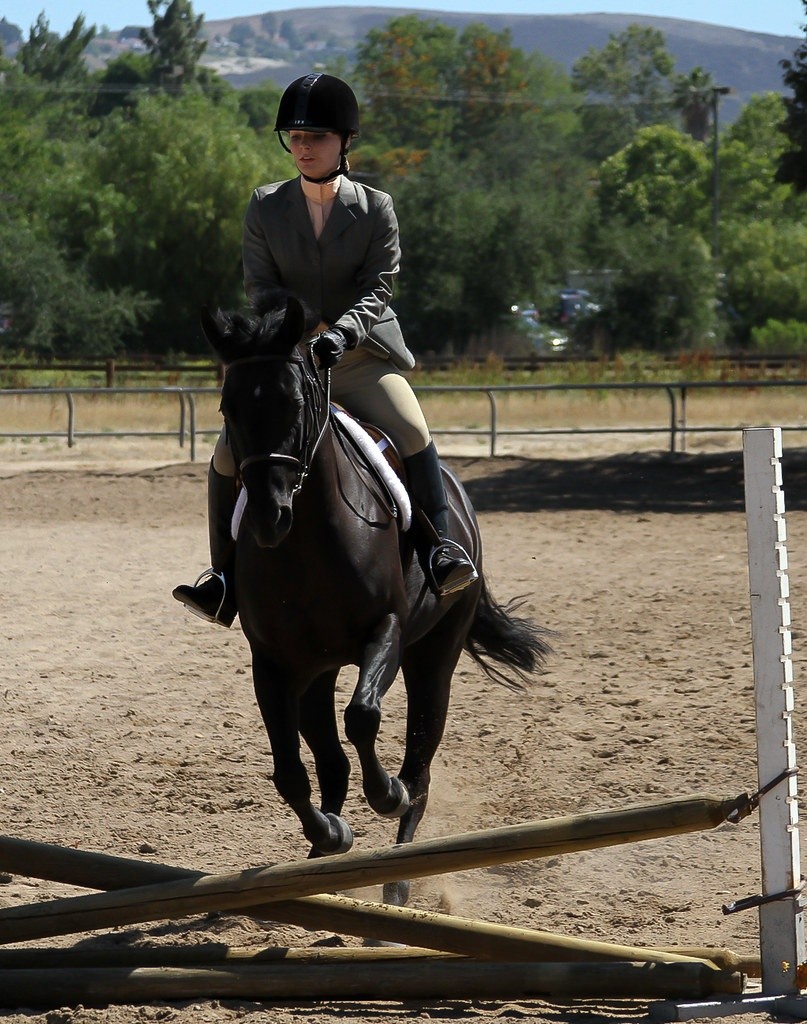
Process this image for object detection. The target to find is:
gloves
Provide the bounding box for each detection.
[314,326,349,370]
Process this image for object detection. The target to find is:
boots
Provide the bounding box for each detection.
[401,434,475,603]
[172,455,240,629]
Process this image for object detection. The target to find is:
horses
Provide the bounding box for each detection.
[197,294,560,947]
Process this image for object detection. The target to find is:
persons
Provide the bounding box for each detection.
[170,73,479,631]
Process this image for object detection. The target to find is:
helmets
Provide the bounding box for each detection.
[273,73,359,137]
